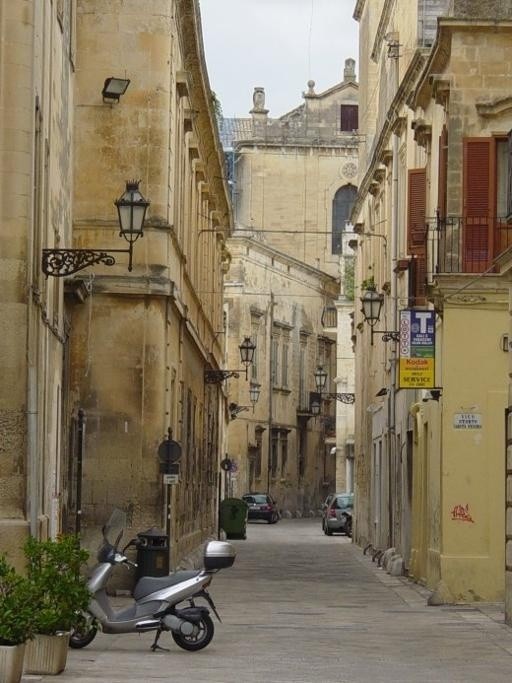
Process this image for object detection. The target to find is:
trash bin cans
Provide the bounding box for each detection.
[221,498,249,539]
[137,526,170,578]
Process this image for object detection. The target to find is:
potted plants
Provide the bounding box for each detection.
[1,528,97,682]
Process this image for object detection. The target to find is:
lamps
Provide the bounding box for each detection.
[42,176,152,280]
[310,363,355,424]
[100,76,131,107]
[359,278,399,348]
[204,332,262,416]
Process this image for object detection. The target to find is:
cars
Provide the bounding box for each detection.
[322,492,353,537]
[242,493,278,524]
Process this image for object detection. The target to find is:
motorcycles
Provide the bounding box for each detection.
[69,525,237,652]
